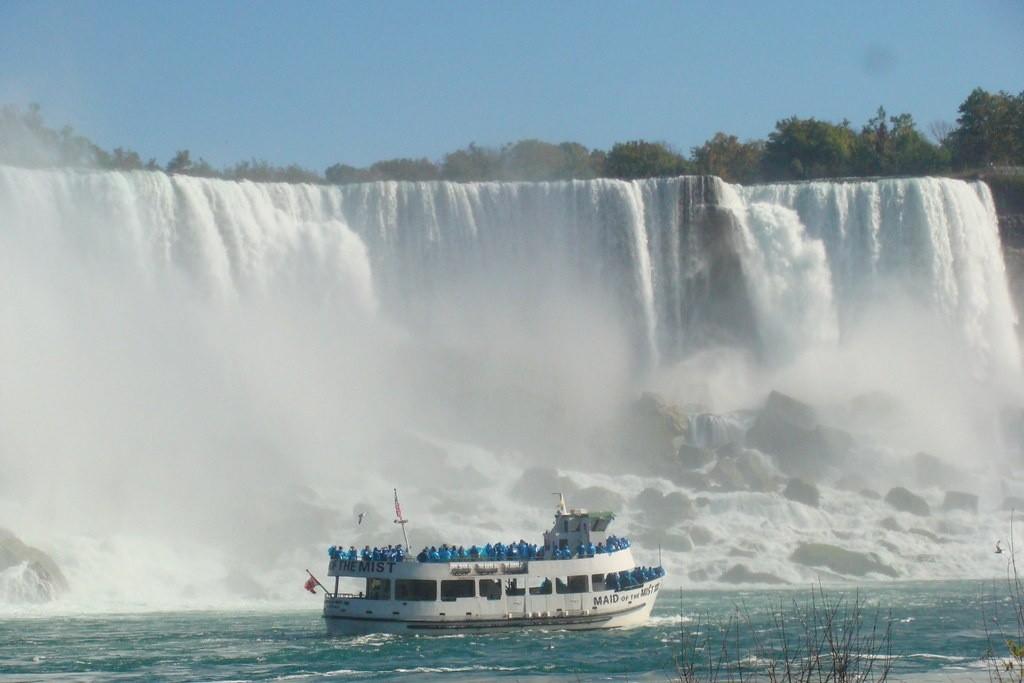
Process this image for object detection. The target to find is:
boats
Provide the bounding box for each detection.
[303,488,665,631]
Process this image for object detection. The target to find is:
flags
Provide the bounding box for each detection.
[304,576,321,594]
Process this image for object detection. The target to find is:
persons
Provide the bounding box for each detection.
[329,535,665,594]
